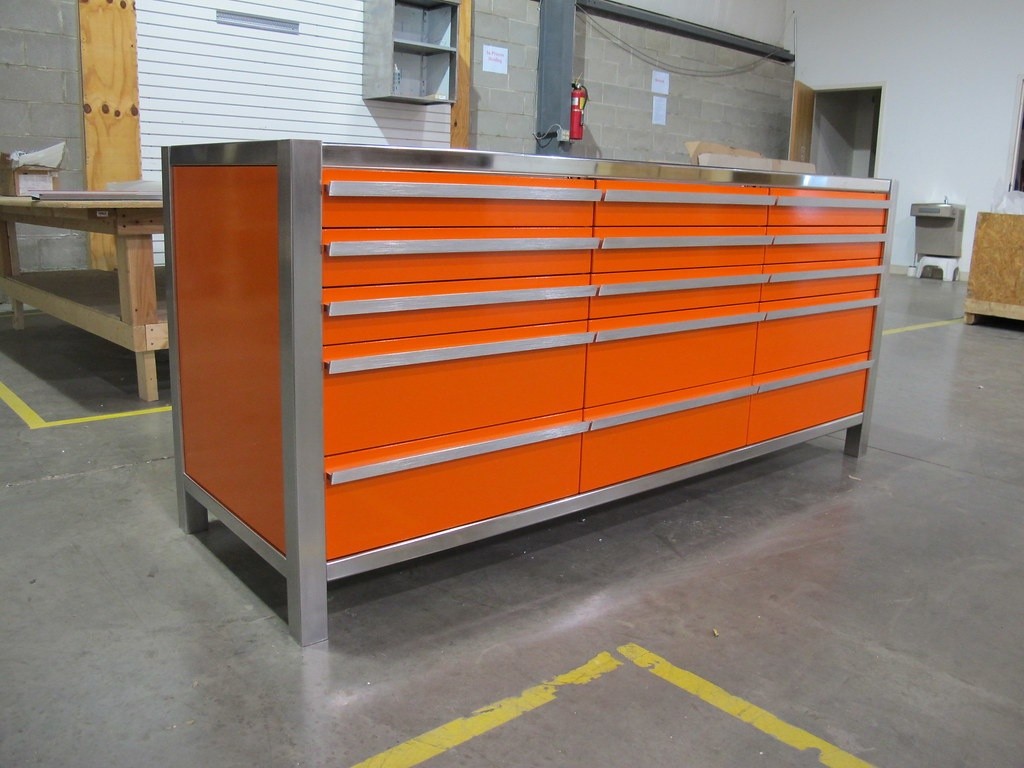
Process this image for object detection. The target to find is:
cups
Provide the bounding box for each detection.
[907,266,916,277]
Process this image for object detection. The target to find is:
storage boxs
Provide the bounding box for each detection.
[0,152,66,196]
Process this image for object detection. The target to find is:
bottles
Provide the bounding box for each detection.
[393,63,400,95]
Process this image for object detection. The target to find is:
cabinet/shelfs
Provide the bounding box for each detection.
[362,0,462,107]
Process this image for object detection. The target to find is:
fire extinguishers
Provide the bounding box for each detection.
[569,73,589,140]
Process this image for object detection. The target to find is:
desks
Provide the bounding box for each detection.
[963,212,1024,325]
[0,195,168,402]
[161,138,900,628]
